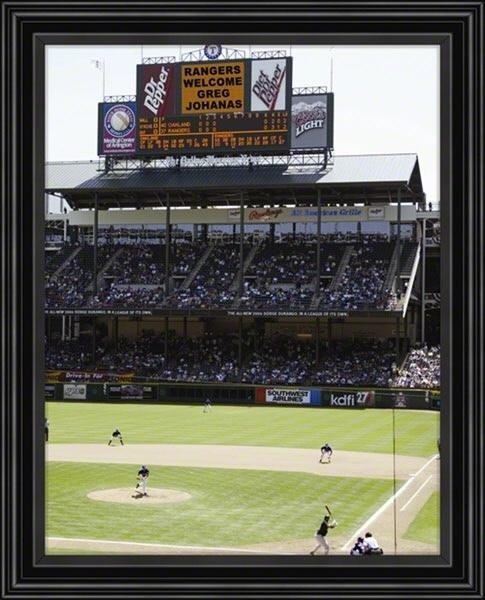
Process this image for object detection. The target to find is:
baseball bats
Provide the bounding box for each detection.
[325,506,334,521]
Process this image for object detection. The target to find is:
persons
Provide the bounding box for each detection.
[136,465,150,495]
[203,397,211,414]
[310,514,337,556]
[363,532,383,555]
[45,418,49,440]
[44,233,402,311]
[350,536,365,556]
[108,428,124,447]
[43,319,440,390]
[319,442,333,462]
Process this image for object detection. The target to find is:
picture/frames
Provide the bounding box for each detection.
[0,0,485,600]
